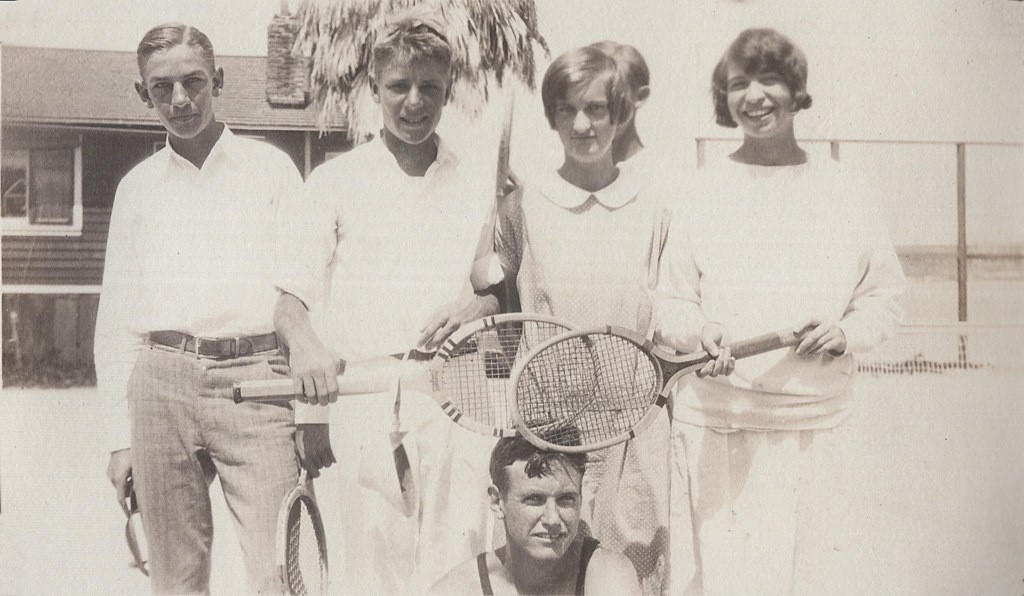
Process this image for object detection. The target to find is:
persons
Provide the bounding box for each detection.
[274,14,503,596]
[648,28,907,596]
[93,23,336,596]
[497,39,699,596]
[425,418,643,596]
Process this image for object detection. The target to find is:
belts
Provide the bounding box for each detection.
[149,330,279,357]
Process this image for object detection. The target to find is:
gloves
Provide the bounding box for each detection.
[294,424,337,478]
